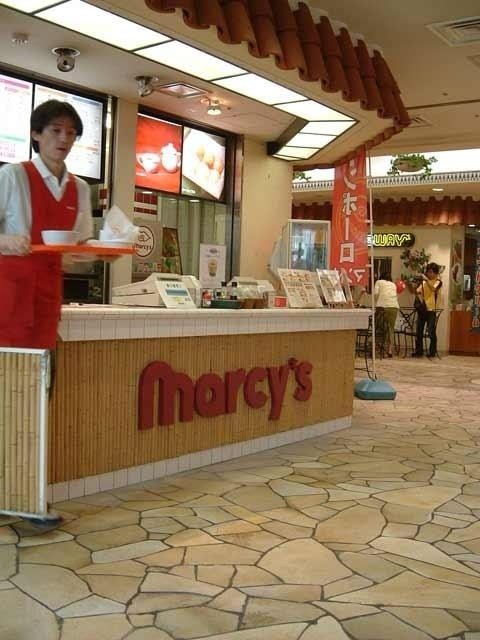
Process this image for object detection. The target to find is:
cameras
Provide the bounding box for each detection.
[413,275,422,284]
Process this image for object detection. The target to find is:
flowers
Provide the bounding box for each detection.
[400,247,431,284]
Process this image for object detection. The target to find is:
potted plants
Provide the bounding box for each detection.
[387,152,438,181]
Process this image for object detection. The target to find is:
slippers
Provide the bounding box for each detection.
[386,350,393,357]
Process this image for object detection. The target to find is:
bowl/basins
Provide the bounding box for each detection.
[40,229,80,246]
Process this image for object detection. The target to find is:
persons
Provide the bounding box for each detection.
[373,273,401,357]
[411,262,443,357]
[1,100,120,525]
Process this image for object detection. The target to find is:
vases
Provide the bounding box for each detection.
[405,281,420,294]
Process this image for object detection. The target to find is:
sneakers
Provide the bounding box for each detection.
[412,351,423,358]
[426,353,436,358]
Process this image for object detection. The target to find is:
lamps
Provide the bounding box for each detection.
[201,96,228,116]
[52,47,80,72]
[135,76,160,98]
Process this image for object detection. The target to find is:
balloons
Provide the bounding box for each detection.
[396,280,406,293]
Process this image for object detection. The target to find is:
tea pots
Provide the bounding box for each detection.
[161,143,181,172]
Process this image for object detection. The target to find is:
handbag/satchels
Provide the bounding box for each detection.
[414,296,426,315]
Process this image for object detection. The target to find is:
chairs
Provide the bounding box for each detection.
[353,305,444,381]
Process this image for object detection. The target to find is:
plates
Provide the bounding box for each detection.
[87,240,136,249]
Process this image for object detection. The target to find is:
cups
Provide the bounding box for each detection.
[208,259,217,277]
[137,153,160,173]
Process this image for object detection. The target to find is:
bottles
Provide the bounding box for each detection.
[220,281,238,300]
